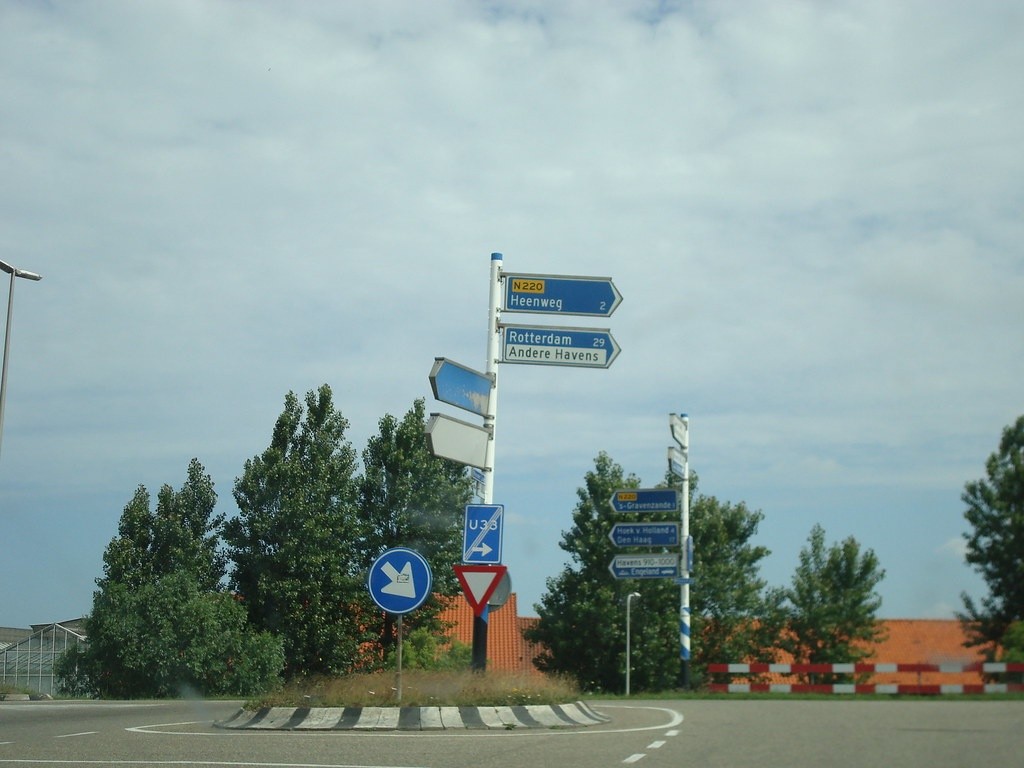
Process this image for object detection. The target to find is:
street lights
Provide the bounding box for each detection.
[625,590,643,697]
[0,258,44,458]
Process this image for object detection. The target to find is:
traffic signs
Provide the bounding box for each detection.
[669,413,687,449]
[607,523,679,548]
[500,325,623,370]
[607,552,680,578]
[609,488,679,513]
[668,447,686,479]
[503,273,624,317]
